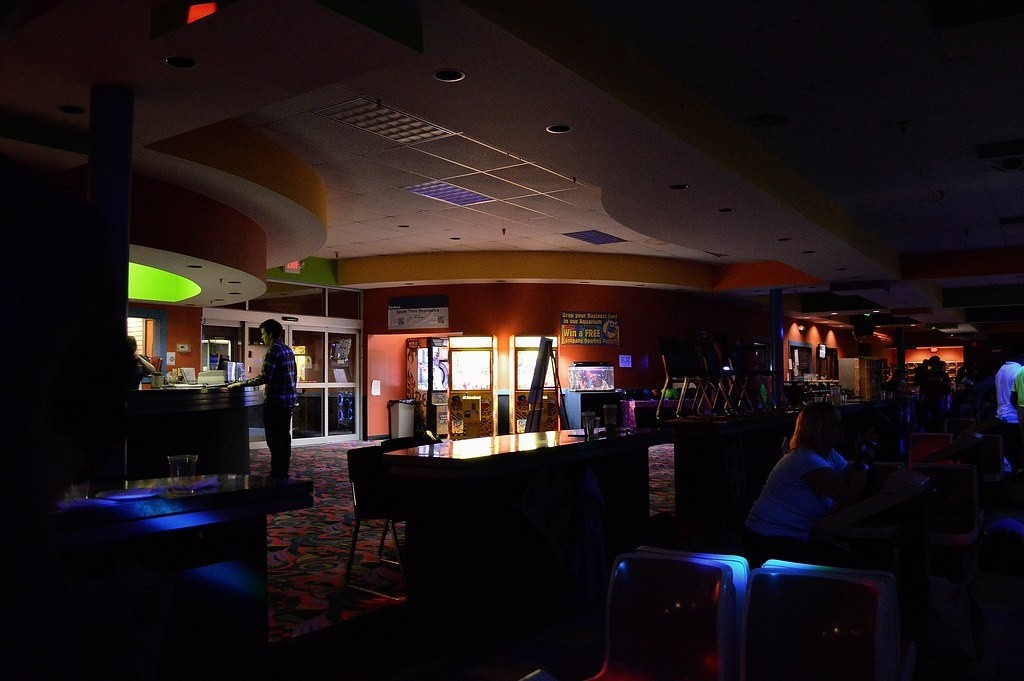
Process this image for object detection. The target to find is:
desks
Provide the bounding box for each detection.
[817,491,904,576]
[659,409,800,526]
[784,378,952,457]
[381,425,673,653]
[0,473,315,681]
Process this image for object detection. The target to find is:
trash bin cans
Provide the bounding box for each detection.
[388,399,416,439]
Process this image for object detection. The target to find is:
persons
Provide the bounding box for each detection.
[741,402,880,566]
[227,319,297,487]
[127,336,156,390]
[887,342,1024,457]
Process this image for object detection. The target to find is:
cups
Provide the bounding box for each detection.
[167,455,199,494]
[603,405,618,440]
[63,478,89,503]
[593,417,600,440]
[880,390,892,400]
[582,412,595,441]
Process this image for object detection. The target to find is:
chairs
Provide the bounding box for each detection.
[655,332,791,420]
[346,436,421,572]
[908,418,1004,548]
[583,545,919,681]
